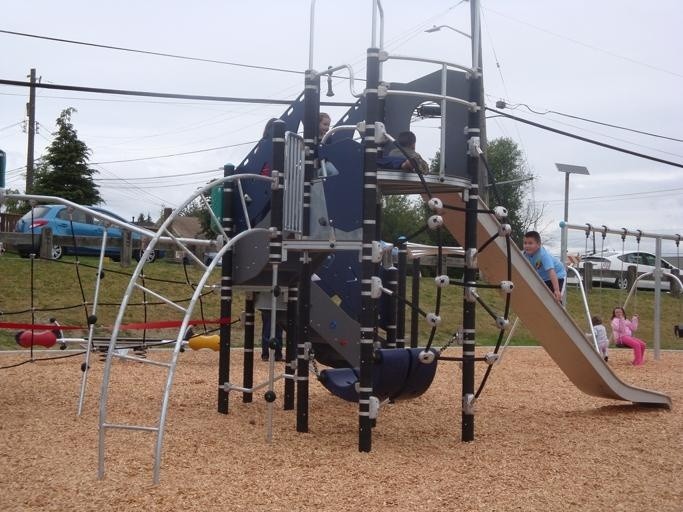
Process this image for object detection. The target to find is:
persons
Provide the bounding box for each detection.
[378,131,429,173]
[522,231,566,305]
[584,307,645,366]
[260,118,279,179]
[318,112,332,144]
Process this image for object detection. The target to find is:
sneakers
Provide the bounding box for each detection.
[412,153,429,175]
[604,356,608,362]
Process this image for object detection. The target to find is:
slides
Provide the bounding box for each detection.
[419,172,672,411]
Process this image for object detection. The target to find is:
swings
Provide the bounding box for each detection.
[585,231,683,349]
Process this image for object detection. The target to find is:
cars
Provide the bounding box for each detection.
[15,204,167,263]
[579,252,683,290]
